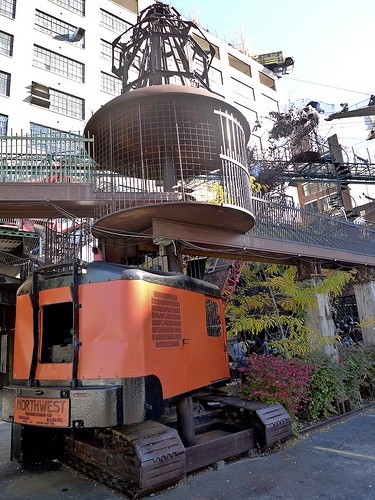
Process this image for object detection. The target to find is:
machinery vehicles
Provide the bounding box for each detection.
[0,259,293,500]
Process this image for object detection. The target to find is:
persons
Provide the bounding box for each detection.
[368,94,375,106]
[305,101,325,114]
[339,103,349,112]
[233,336,247,363]
